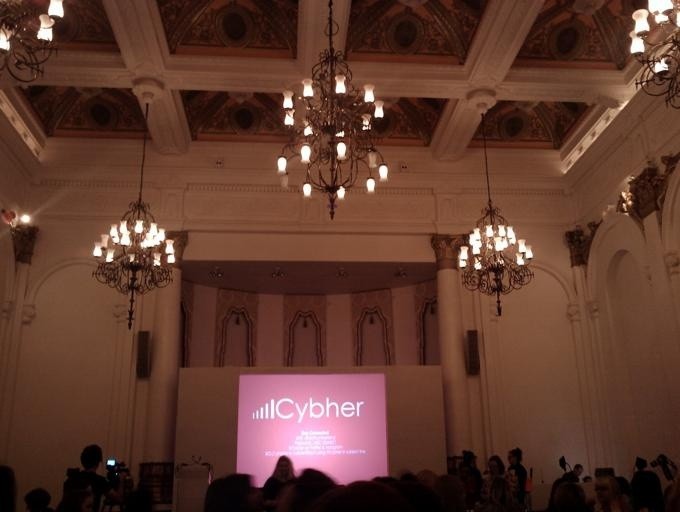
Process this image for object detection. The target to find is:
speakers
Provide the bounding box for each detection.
[135,330,149,380]
[465,329,479,374]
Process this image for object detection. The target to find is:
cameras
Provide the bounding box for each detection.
[650,454,669,468]
[105,459,130,473]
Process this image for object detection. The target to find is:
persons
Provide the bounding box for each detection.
[0,440,680,510]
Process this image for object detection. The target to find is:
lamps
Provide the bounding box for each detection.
[0,1,66,85]
[458,90,535,317]
[628,0,680,112]
[88,77,179,331]
[273,3,391,222]
